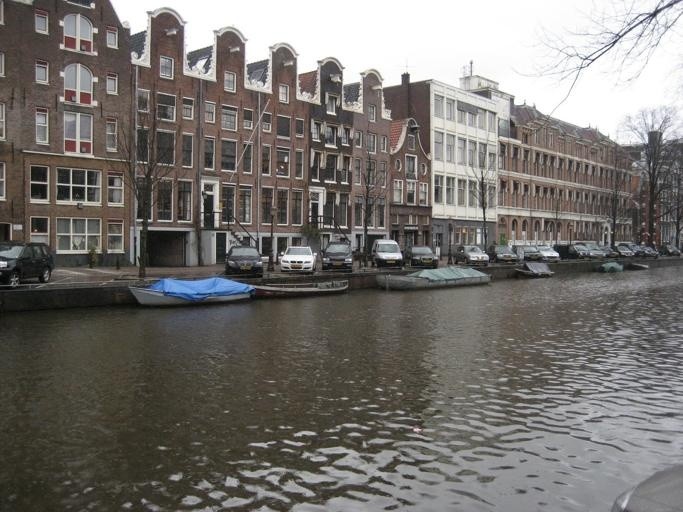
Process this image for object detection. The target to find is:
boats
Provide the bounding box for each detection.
[245,278,349,299]
[513,262,555,278]
[126,273,255,307]
[594,261,649,274]
[374,265,490,290]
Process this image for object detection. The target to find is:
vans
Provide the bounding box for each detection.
[370,238,403,268]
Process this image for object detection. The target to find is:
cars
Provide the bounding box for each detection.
[224,245,264,277]
[535,246,559,262]
[401,246,437,267]
[553,241,681,259]
[453,244,489,266]
[279,245,317,274]
[484,244,516,262]
[510,245,543,262]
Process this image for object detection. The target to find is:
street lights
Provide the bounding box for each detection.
[568,223,573,244]
[266,206,278,272]
[446,220,453,265]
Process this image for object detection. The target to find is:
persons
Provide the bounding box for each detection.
[492,240,497,244]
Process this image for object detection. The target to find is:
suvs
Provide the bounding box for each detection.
[0,238,53,290]
[320,241,354,272]
